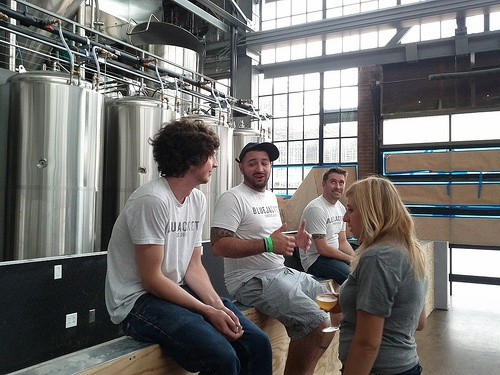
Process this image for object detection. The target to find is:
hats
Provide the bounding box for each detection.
[235,142,279,163]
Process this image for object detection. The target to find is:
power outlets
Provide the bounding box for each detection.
[66,313,77,328]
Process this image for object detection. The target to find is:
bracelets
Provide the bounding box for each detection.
[263,236,273,252]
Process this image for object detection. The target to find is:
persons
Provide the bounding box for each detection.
[298,167,357,286]
[210,142,341,375]
[339,176,424,375]
[105,120,275,375]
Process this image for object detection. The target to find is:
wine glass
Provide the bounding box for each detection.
[316,295,339,333]
